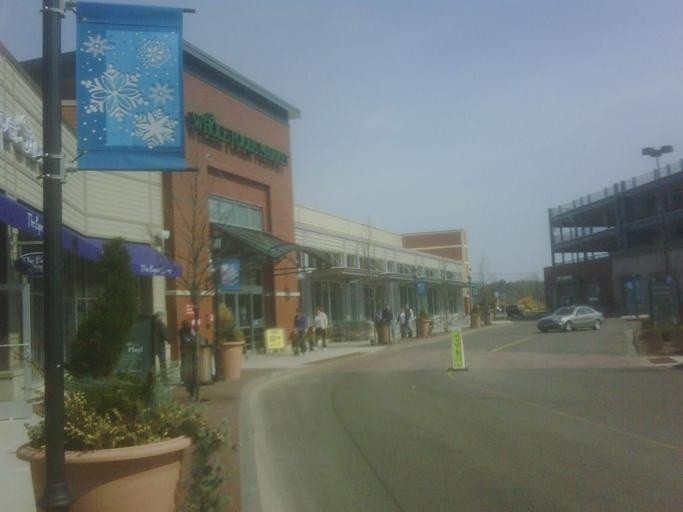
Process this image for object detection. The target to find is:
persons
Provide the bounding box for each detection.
[288,328,302,356]
[314,307,328,347]
[152,310,172,382]
[374,302,416,339]
[304,326,321,351]
[179,319,196,380]
[293,307,308,354]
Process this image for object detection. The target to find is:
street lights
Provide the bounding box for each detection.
[642,146,674,281]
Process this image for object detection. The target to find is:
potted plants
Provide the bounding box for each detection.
[375,304,482,345]
[15,232,244,511]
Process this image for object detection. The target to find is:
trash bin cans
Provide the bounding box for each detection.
[377,317,392,344]
[196,338,215,386]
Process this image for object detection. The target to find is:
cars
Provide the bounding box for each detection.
[332,321,379,346]
[538,305,603,333]
